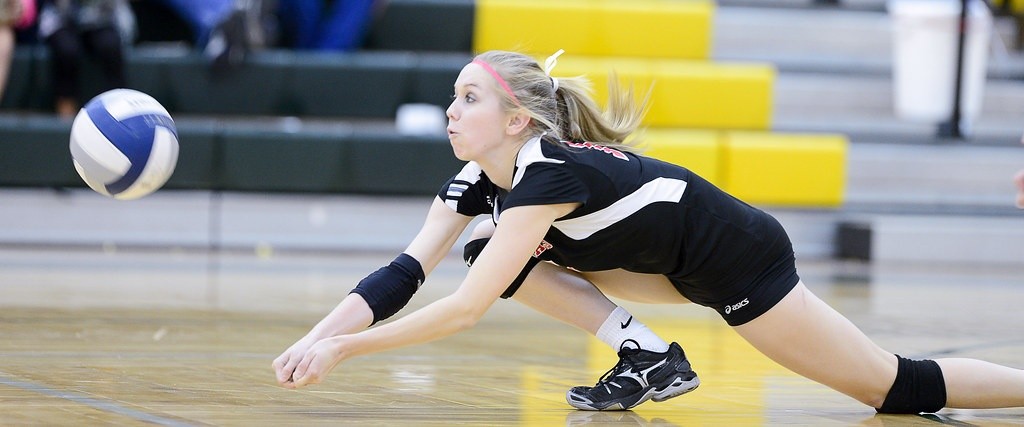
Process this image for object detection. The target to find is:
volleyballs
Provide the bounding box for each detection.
[67,84,182,203]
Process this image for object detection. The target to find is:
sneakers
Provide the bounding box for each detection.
[566,342,699,410]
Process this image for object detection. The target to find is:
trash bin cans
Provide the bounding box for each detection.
[886,0,993,139]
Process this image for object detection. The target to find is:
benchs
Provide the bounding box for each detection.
[0,0,1021,269]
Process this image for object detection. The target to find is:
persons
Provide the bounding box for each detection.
[272,50,1024,414]
[0,0,37,107]
[37,1,146,121]
[295,1,377,51]
[174,0,282,86]
